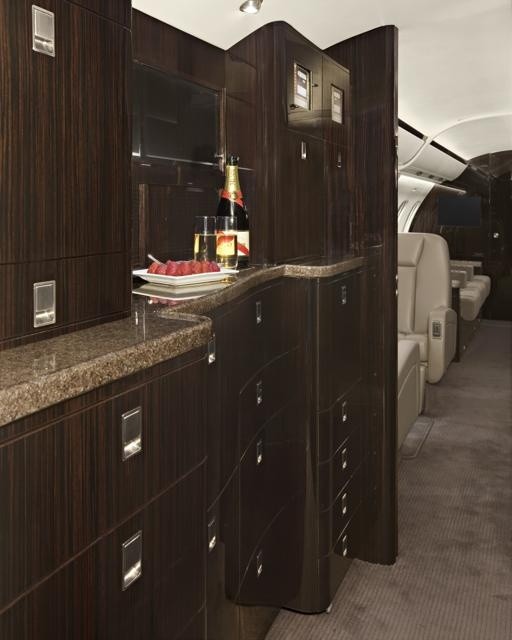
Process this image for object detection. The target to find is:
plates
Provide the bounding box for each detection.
[131,267,241,286]
[131,282,230,301]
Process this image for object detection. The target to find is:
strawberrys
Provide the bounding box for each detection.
[167,262,179,276]
[180,262,192,275]
[156,263,167,275]
[191,261,202,274]
[202,261,208,272]
[208,262,220,272]
[147,262,159,274]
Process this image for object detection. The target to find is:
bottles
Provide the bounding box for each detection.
[215,155,250,267]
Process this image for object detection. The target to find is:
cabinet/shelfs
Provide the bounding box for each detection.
[0,1,401,639]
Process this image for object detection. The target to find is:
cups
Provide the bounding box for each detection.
[191,214,216,261]
[216,214,238,284]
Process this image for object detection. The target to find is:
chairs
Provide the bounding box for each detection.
[448,259,492,363]
[397,232,457,383]
[396,340,422,454]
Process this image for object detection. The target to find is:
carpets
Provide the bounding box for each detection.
[262,319,512,640]
